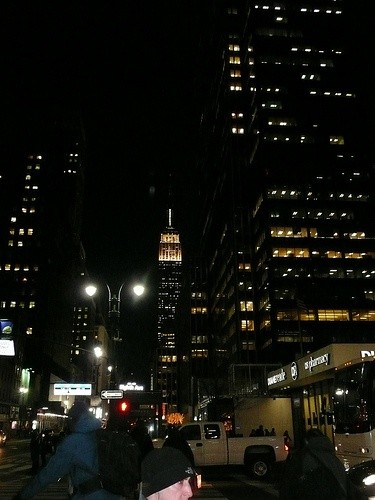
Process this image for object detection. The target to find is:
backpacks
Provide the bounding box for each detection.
[73,430,139,496]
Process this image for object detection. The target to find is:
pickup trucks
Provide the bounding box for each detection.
[151,421,290,483]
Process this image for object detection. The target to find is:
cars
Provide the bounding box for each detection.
[0,428,8,448]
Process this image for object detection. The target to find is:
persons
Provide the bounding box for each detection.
[13,402,124,500]
[27,430,52,468]
[135,420,193,460]
[249,425,292,443]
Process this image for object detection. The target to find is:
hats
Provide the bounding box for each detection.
[70,402,86,421]
[141,446,194,496]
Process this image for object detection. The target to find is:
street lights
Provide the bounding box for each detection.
[83,280,147,390]
